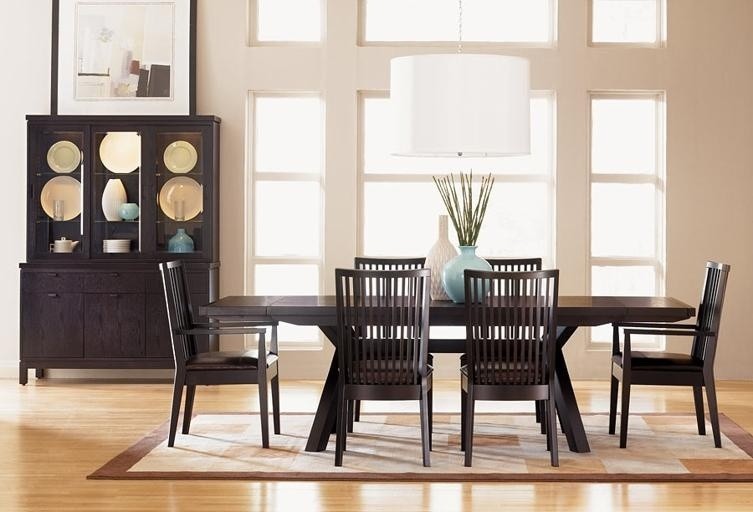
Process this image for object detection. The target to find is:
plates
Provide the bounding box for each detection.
[163,140,198,173]
[98,134,139,172]
[46,140,80,173]
[40,175,80,222]
[101,238,132,254]
[159,176,202,221]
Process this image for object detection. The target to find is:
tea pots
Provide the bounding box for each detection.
[48,237,78,253]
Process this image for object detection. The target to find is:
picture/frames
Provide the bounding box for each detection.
[49,0,197,122]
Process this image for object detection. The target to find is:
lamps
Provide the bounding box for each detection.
[385,0,533,163]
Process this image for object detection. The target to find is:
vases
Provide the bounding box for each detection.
[99,177,128,222]
[164,227,194,253]
[441,244,494,306]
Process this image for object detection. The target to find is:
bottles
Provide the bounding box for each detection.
[166,228,195,253]
[100,179,126,221]
[118,202,138,221]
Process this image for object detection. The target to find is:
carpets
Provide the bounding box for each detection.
[87,407,753,483]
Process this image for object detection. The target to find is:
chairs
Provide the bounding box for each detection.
[158,255,281,451]
[334,267,441,468]
[458,266,564,468]
[458,256,547,435]
[604,258,732,450]
[345,255,435,434]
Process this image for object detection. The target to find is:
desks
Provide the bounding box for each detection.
[195,293,697,455]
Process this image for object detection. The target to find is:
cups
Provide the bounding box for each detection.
[51,199,65,223]
[173,199,185,220]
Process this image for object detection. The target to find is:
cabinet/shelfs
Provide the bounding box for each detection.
[18,262,222,387]
[21,114,223,266]
